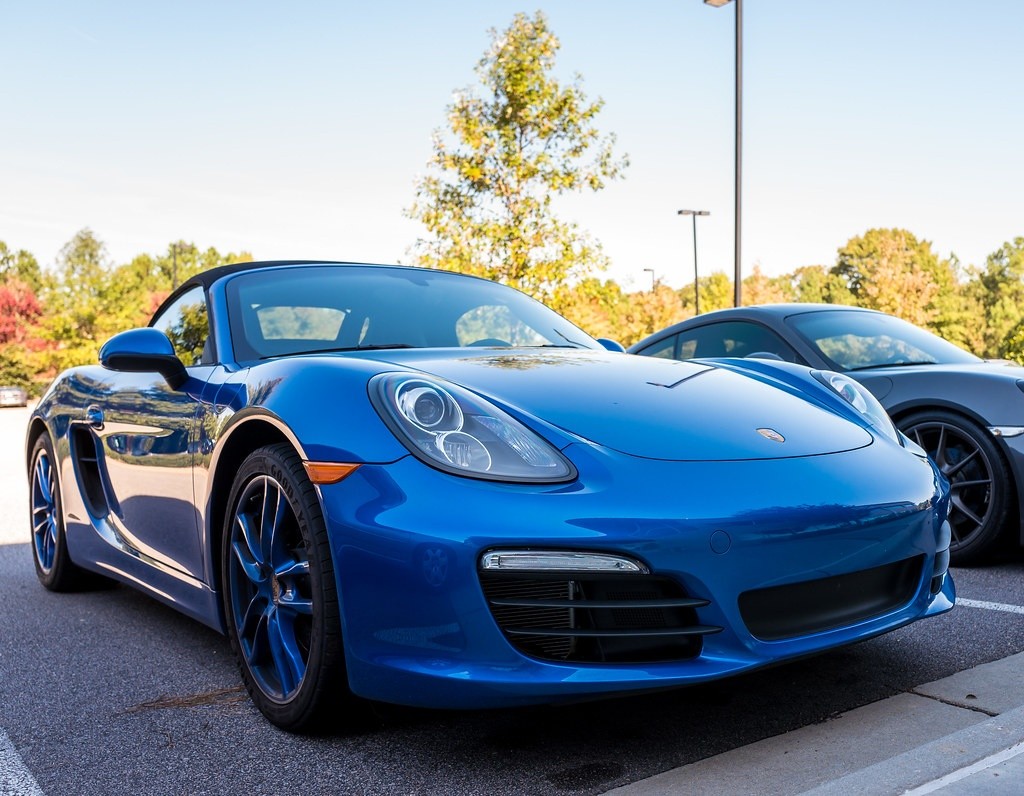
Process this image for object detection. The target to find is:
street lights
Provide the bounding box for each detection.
[644,268,655,293]
[702,0,744,309]
[676,208,718,316]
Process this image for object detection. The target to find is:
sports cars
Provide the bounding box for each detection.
[27,257,958,734]
[626,300,1024,571]
